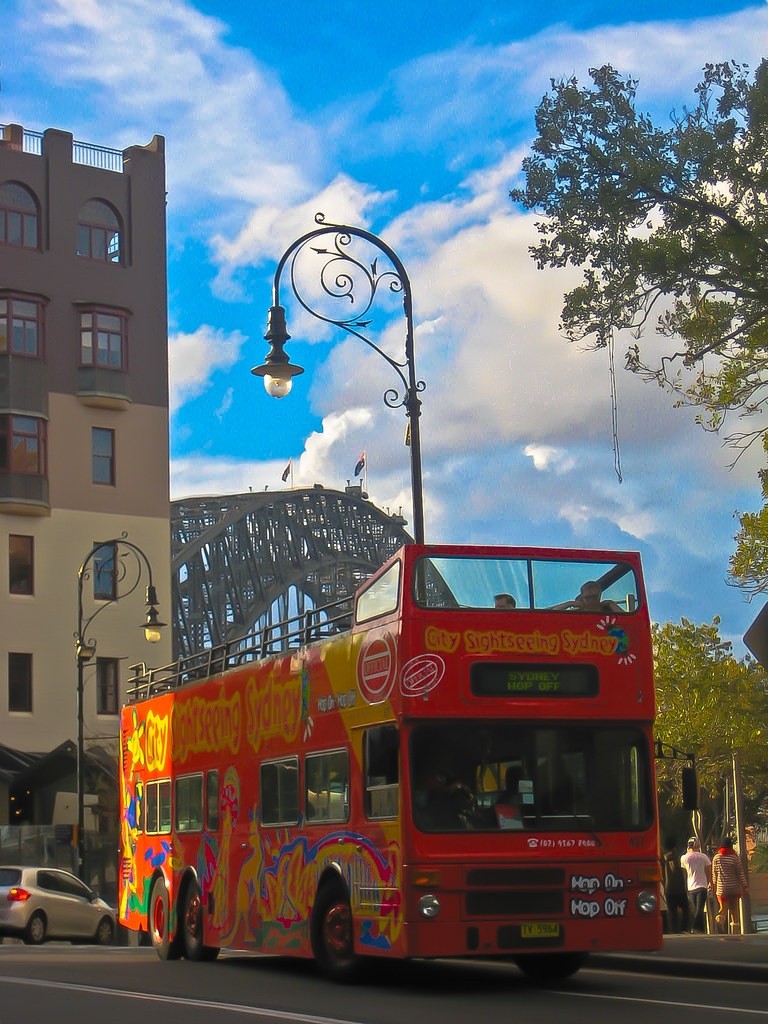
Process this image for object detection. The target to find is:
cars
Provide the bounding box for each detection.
[0,865,117,945]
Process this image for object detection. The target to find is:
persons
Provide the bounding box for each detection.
[544,581,627,612]
[680,837,712,933]
[711,837,750,931]
[414,758,601,831]
[664,838,688,932]
[494,594,516,609]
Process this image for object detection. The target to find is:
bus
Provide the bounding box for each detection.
[117,543,666,979]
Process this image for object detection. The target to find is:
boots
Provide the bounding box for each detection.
[730,922,740,935]
[715,915,725,934]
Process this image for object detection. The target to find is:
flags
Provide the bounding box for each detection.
[282,460,291,481]
[354,451,365,476]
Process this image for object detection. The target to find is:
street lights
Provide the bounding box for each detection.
[252,212,430,606]
[72,531,167,881]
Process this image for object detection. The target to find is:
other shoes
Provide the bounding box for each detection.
[692,929,704,934]
[682,929,690,934]
